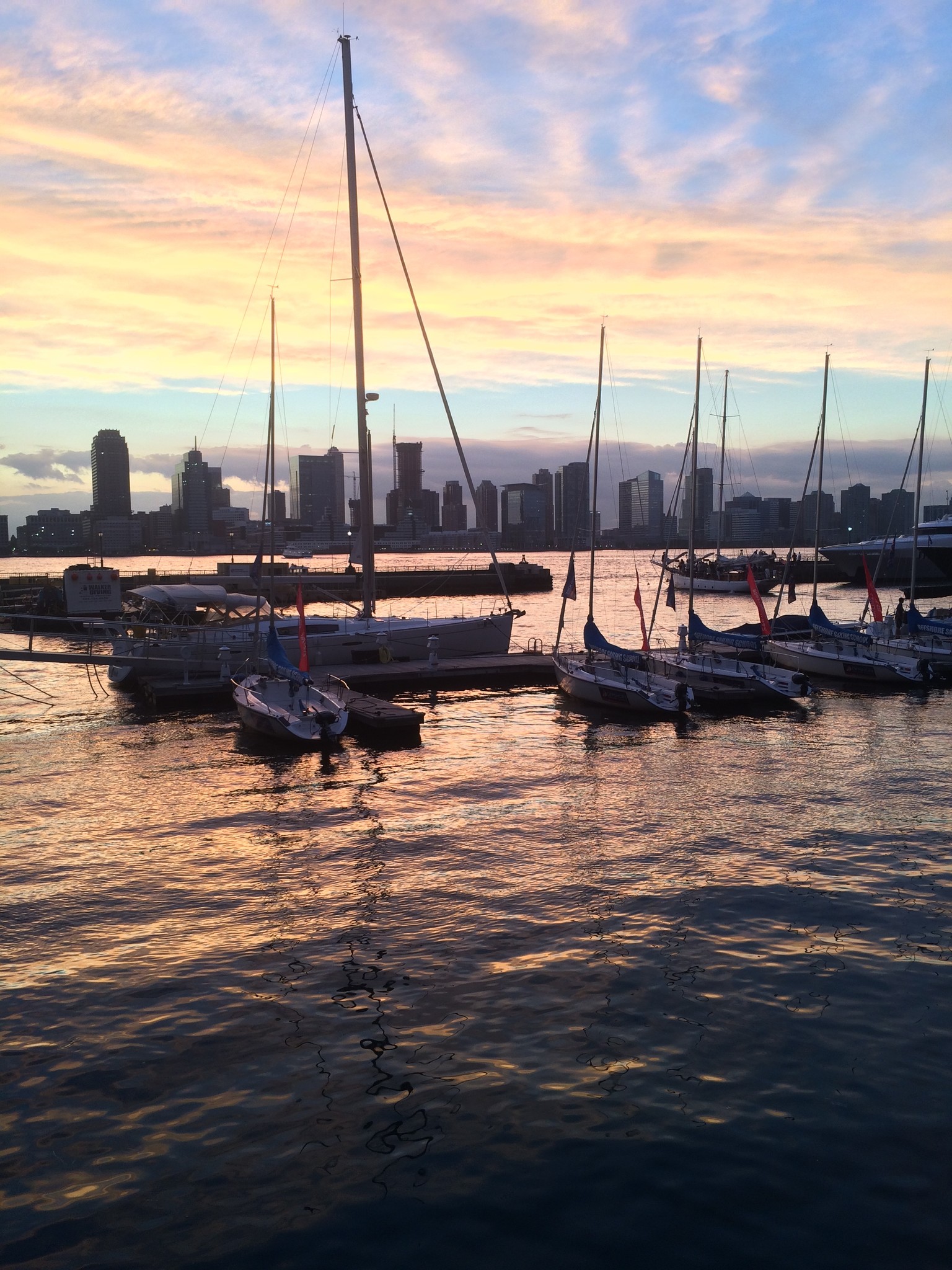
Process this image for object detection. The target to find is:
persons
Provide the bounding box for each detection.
[662,547,802,579]
[894,597,905,639]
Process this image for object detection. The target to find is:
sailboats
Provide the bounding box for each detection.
[547,328,948,713]
[1,29,559,757]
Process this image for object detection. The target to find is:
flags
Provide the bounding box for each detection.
[744,562,770,635]
[886,532,896,570]
[294,582,308,670]
[666,573,676,611]
[250,544,262,586]
[787,562,797,604]
[561,562,576,600]
[861,553,883,625]
[633,571,650,651]
